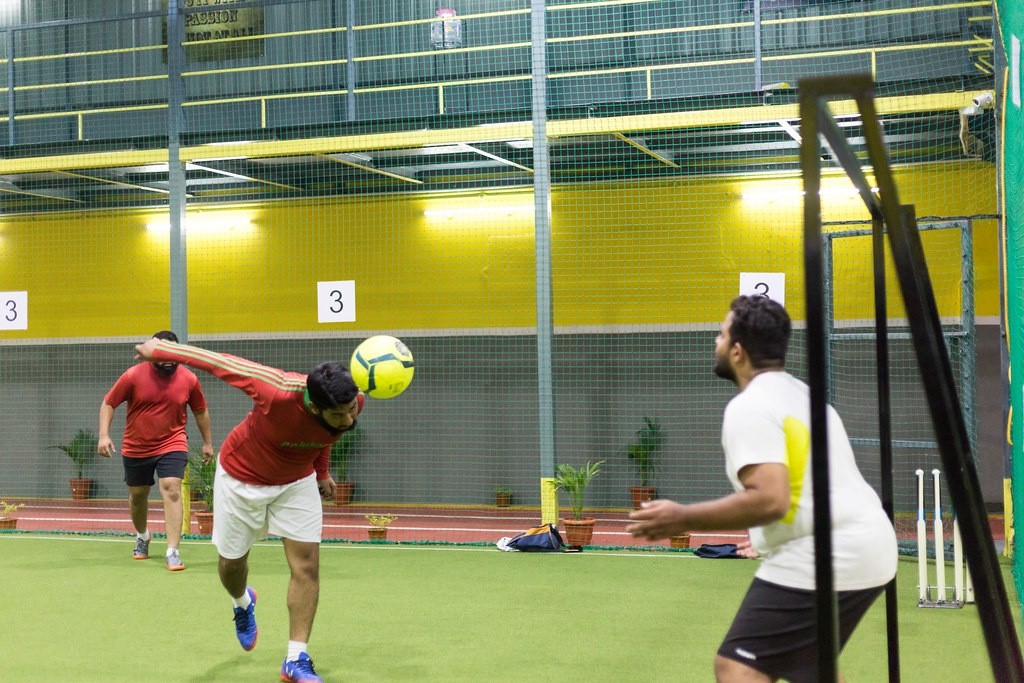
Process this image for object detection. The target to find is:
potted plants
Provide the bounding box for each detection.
[671,529,692,548]
[184,443,217,536]
[617,418,664,513]
[329,425,365,505]
[361,512,397,539]
[551,460,606,550]
[0,499,25,529]
[45,428,98,500]
[497,484,515,507]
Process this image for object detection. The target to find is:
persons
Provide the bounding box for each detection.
[135,337,365,683]
[625,292,898,683]
[97,331,214,570]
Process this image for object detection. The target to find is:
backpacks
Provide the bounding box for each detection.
[506,524,583,552]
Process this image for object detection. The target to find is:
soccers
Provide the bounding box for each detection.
[348,334,415,400]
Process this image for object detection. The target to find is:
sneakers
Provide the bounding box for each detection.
[280,652,323,683]
[133,535,151,559]
[233,587,257,651]
[166,552,184,570]
